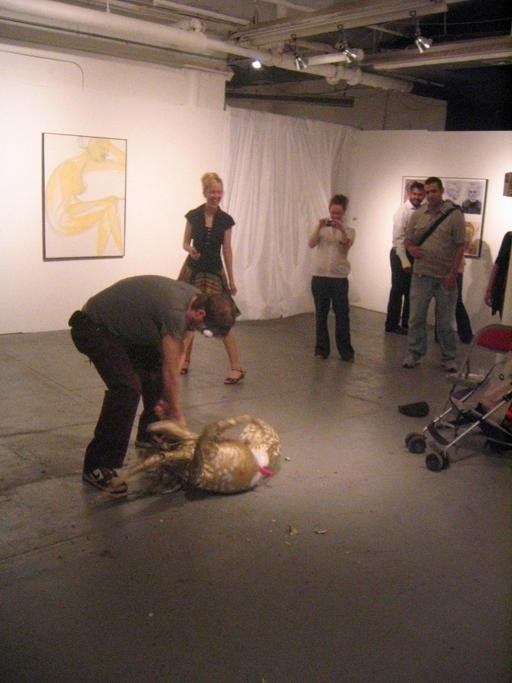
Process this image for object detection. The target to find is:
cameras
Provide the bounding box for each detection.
[326,219,335,226]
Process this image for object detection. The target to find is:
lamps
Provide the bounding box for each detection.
[291,10,435,73]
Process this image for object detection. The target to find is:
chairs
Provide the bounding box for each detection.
[441,323,510,438]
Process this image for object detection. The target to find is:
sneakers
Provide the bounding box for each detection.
[134,431,180,449]
[403,355,416,368]
[83,468,127,494]
[441,361,457,372]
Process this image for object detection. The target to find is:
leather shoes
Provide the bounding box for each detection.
[392,328,407,336]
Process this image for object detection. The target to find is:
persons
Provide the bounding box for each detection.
[309,192,356,364]
[176,170,245,387]
[435,194,473,347]
[67,272,234,497]
[461,182,482,214]
[400,177,465,372]
[384,181,427,335]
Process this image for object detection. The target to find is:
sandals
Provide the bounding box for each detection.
[181,360,190,375]
[224,368,244,384]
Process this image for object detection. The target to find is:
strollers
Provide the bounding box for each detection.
[406,322,512,473]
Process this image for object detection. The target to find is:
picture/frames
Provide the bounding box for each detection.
[398,175,488,259]
